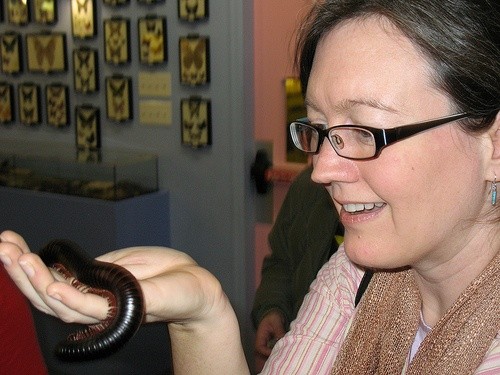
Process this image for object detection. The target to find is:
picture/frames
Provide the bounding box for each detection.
[104,73,134,125]
[16,80,42,129]
[137,12,168,68]
[74,102,102,148]
[178,33,211,88]
[0,80,16,127]
[6,0,32,28]
[72,44,100,95]
[177,0,210,26]
[0,30,24,78]
[25,29,69,75]
[180,94,213,148]
[103,15,133,67]
[45,80,71,130]
[69,0,99,42]
[32,0,59,27]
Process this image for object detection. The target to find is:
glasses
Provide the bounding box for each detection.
[288,112,468,161]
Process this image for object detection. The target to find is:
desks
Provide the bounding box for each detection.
[0,186,171,375]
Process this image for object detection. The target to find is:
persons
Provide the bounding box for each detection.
[0,0,499,375]
